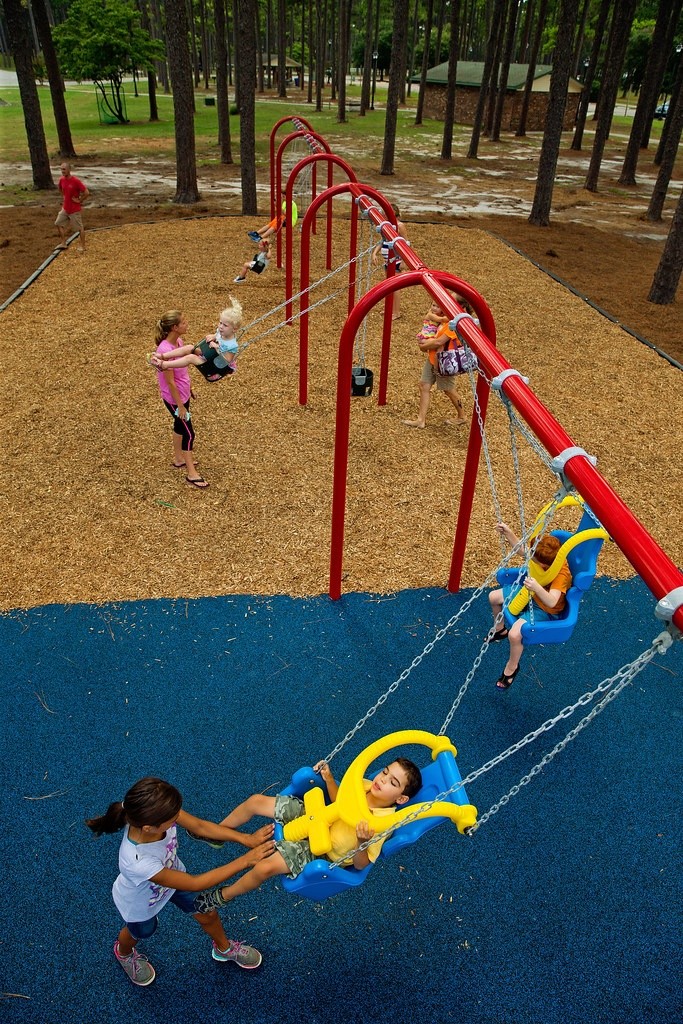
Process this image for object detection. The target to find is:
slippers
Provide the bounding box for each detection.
[172,461,198,466]
[186,475,210,487]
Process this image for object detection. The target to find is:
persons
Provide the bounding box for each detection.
[153,309,211,488]
[483,534,574,691]
[232,237,272,284]
[247,189,298,242]
[186,756,431,915]
[145,292,243,383]
[371,203,409,322]
[84,777,263,986]
[399,291,475,430]
[54,162,90,252]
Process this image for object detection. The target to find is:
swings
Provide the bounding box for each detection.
[250,153,316,274]
[191,238,395,383]
[462,341,616,646]
[274,487,674,904]
[350,215,375,398]
[282,127,297,227]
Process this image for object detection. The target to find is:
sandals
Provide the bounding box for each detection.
[484,624,509,642]
[495,663,520,687]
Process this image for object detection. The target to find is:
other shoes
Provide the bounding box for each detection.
[248,231,259,239]
[151,352,163,360]
[447,417,466,425]
[400,419,425,429]
[78,247,86,252]
[147,354,164,374]
[251,236,263,243]
[54,244,68,249]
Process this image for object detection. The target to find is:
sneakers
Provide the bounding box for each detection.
[185,829,224,849]
[211,939,262,969]
[234,276,245,282]
[114,939,156,986]
[193,885,233,914]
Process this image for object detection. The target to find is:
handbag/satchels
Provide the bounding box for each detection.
[437,339,479,378]
